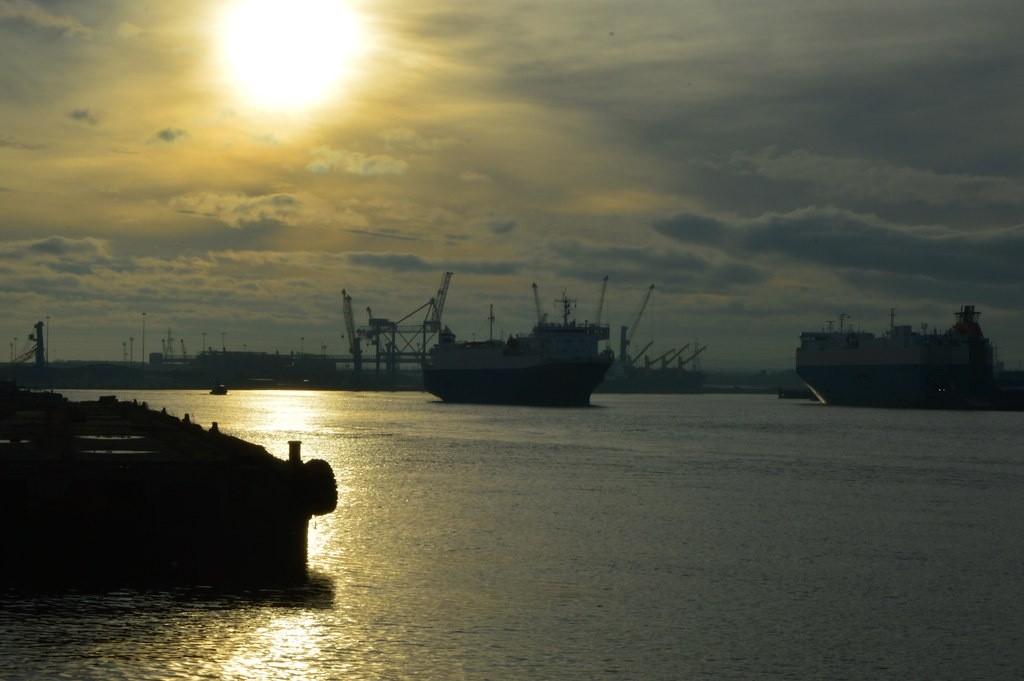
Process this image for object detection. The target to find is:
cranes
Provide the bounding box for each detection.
[338,274,707,380]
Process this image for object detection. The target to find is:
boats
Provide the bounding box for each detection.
[419,274,616,409]
[794,303,997,407]
[210,385,228,395]
